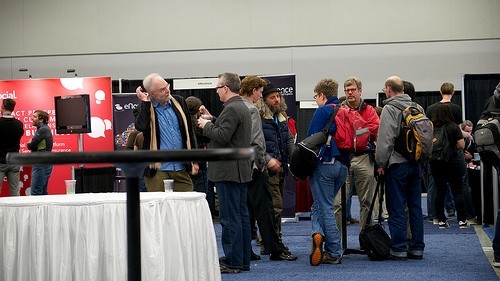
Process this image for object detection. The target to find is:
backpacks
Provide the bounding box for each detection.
[388,100,433,161]
[472,111,500,175]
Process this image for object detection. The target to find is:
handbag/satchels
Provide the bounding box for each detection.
[290,132,327,180]
[359,224,391,261]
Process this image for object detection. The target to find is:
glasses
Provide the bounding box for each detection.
[152,83,170,93]
[344,89,360,93]
[313,92,326,99]
[216,85,230,90]
[382,87,391,91]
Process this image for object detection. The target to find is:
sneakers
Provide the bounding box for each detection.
[322,252,342,264]
[309,233,323,266]
[439,220,450,228]
[459,220,470,228]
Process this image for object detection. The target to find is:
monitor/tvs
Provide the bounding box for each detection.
[54,95,90,134]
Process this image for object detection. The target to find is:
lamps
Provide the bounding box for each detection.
[67,69,77,77]
[19,68,32,79]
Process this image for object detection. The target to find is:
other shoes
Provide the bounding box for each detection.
[429,219,433,222]
[433,218,439,225]
[385,253,407,260]
[493,256,500,266]
[407,252,423,259]
[250,235,298,260]
[448,209,455,217]
[468,219,478,224]
[219,262,241,273]
[423,216,431,220]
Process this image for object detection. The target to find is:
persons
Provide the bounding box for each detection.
[198,72,253,272]
[132,74,215,211]
[27,110,53,195]
[334,78,380,249]
[256,83,294,256]
[460,120,477,163]
[0,98,24,197]
[375,76,425,260]
[238,75,298,261]
[424,83,471,229]
[487,82,500,266]
[305,79,351,263]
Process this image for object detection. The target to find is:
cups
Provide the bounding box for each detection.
[65,180,76,194]
[474,153,480,159]
[163,179,174,192]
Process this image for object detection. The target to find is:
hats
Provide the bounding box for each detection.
[262,84,281,97]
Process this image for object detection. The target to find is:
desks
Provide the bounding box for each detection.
[0,192,223,281]
